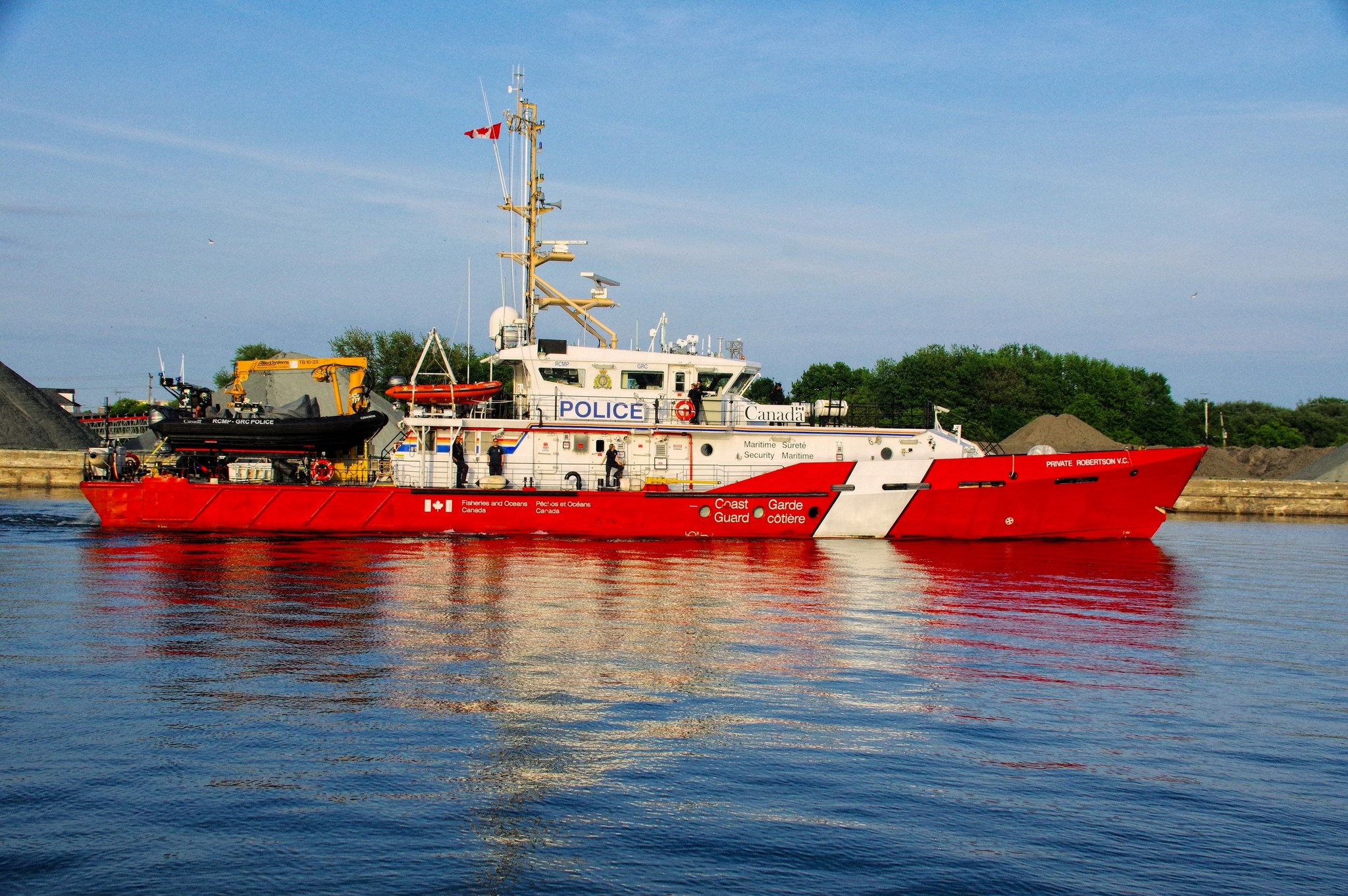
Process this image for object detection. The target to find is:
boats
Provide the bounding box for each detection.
[77,66,1209,545]
[384,376,505,407]
[143,400,387,461]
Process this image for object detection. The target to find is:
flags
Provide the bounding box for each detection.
[464,122,502,139]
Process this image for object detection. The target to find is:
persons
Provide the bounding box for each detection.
[769,383,783,426]
[195,403,203,419]
[687,383,697,424]
[449,435,469,489]
[600,444,625,488]
[691,379,708,425]
[486,438,505,476]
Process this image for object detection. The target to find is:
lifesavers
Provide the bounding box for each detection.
[311,460,334,481]
[675,401,695,421]
[125,452,139,469]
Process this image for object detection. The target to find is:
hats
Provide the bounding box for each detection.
[691,383,696,386]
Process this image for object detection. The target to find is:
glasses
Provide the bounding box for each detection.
[697,383,701,385]
